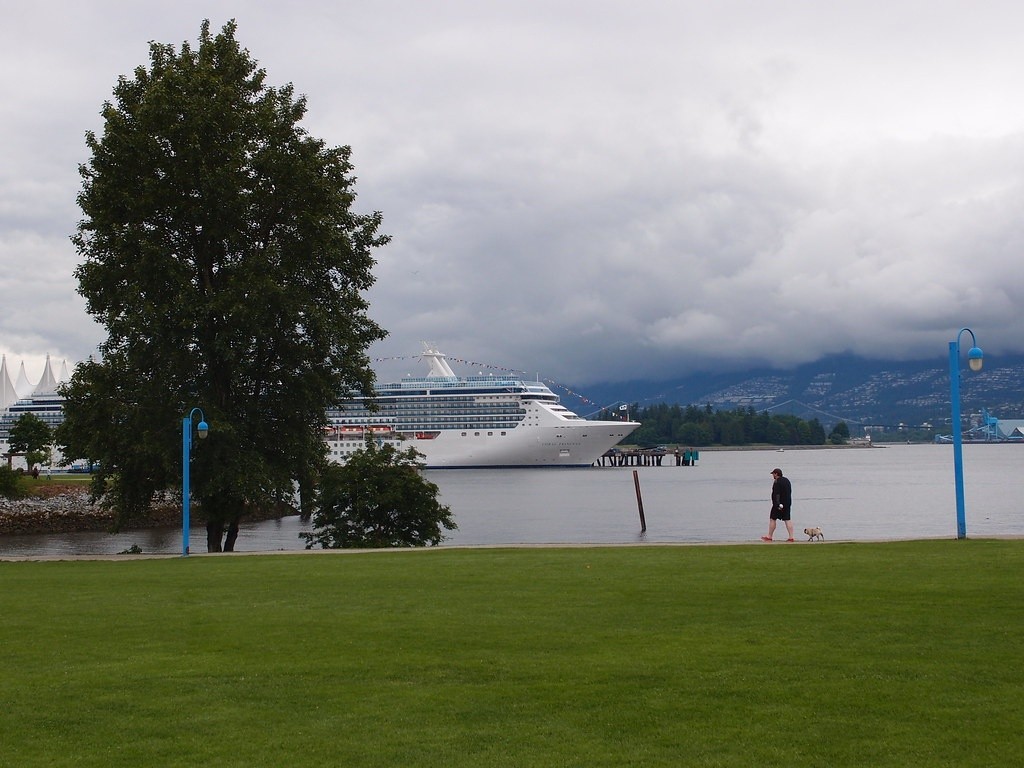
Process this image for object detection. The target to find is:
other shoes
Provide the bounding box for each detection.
[761,536,772,541]
[788,539,794,542]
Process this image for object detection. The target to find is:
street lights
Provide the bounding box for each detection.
[948,328,984,539]
[182,407,209,555]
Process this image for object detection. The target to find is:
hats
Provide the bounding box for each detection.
[770,469,782,474]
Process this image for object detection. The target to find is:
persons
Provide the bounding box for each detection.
[32,468,39,479]
[46,467,51,480]
[760,468,795,542]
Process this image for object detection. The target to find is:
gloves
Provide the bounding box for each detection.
[777,508,785,515]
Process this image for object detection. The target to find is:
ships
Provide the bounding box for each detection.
[1,339,642,473]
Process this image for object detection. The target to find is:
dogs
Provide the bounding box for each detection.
[803,526,825,542]
